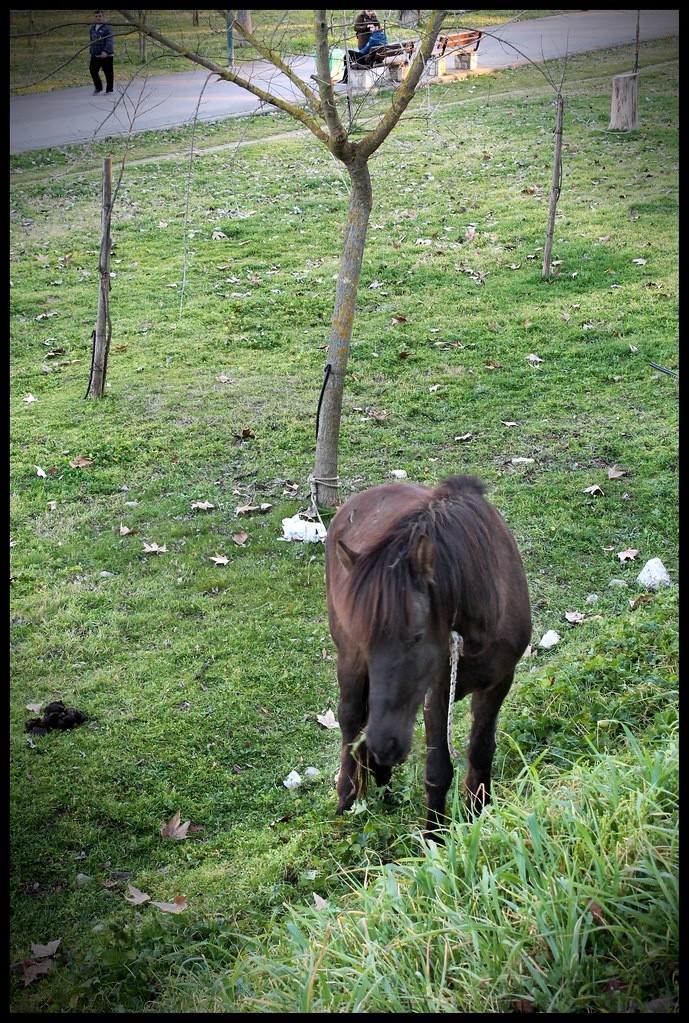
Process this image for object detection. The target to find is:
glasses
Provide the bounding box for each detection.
[94,15,102,18]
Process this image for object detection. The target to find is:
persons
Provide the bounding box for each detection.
[335,10,389,85]
[88,10,115,95]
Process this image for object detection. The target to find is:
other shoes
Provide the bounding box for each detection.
[93,91,102,96]
[104,91,113,95]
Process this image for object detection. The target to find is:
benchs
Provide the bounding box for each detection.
[425,29,486,76]
[344,40,416,89]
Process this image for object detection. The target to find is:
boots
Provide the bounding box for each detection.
[333,75,348,86]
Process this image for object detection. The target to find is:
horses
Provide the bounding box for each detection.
[322,472,532,848]
